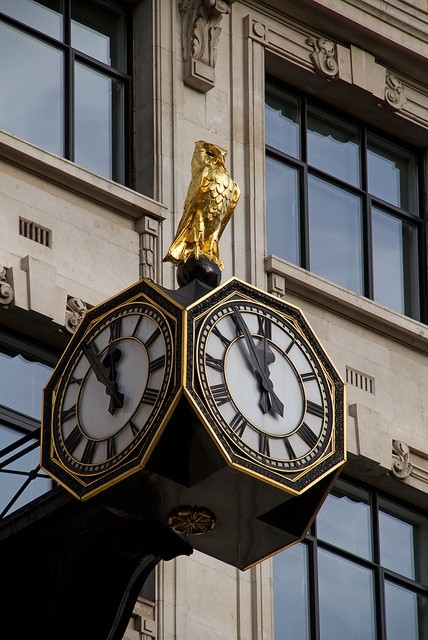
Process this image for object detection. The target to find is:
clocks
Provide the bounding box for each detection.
[193,297,334,472]
[50,300,174,477]
